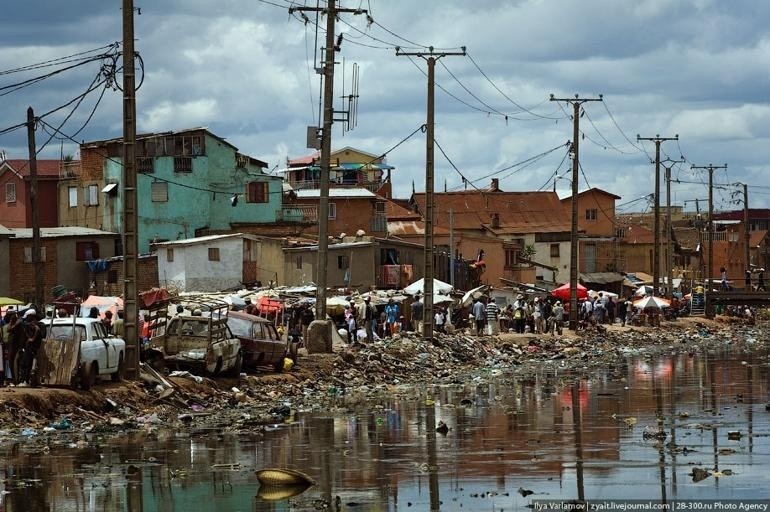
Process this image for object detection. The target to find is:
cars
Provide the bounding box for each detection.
[35,317,127,386]
[217,313,288,375]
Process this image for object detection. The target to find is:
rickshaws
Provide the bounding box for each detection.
[32,302,84,389]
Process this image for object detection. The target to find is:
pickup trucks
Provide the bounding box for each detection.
[143,314,244,379]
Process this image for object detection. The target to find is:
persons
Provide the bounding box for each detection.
[754,267,767,292]
[0,304,203,389]
[719,305,759,319]
[242,292,689,348]
[285,319,303,366]
[719,264,730,291]
[692,282,704,308]
[743,269,752,294]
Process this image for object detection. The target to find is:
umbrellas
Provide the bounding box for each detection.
[359,275,488,308]
[547,280,620,302]
